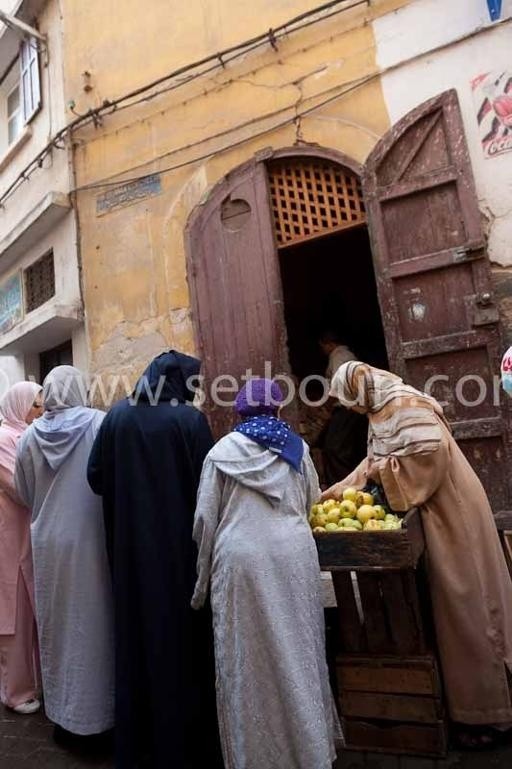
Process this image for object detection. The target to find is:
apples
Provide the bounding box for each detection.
[309,488,399,532]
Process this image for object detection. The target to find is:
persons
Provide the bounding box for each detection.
[191,378,347,769]
[0,381,43,714]
[86,349,214,769]
[14,365,115,762]
[322,360,512,746]
[318,334,358,413]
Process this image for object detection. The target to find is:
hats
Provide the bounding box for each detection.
[234,379,283,416]
